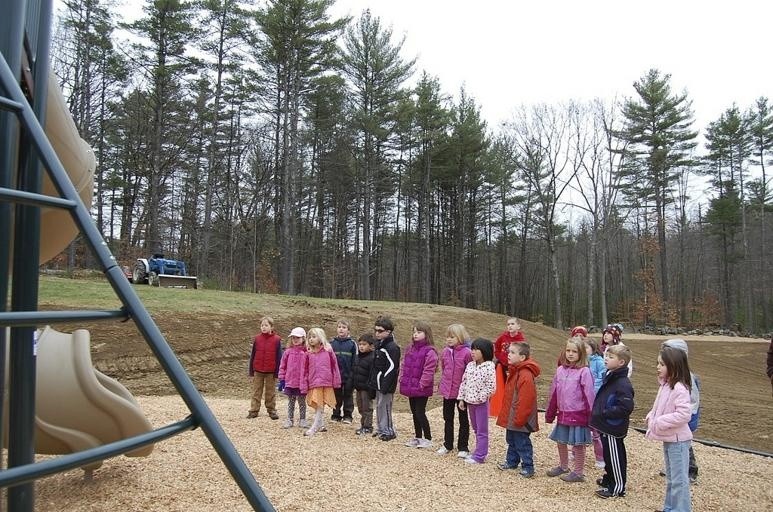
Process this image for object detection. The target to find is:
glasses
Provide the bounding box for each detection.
[374,328,386,334]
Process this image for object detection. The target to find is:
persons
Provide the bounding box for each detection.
[543,338,597,482]
[398,323,439,449]
[556,326,588,367]
[456,337,497,465]
[585,345,634,498]
[299,328,342,436]
[584,340,606,468]
[598,322,632,379]
[433,324,472,457]
[366,319,401,441]
[644,346,694,512]
[657,338,701,485]
[493,317,527,383]
[330,320,357,424]
[351,334,378,435]
[494,340,538,476]
[276,326,312,428]
[246,315,282,420]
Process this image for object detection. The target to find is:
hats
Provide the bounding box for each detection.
[602,323,623,345]
[662,339,688,356]
[571,327,587,337]
[287,327,306,338]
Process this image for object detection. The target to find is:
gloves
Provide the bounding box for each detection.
[278,381,285,391]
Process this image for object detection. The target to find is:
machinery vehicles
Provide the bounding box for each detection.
[122,253,203,289]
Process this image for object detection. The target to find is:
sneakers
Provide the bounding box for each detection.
[560,471,585,482]
[497,463,518,470]
[596,469,612,498]
[404,437,485,464]
[283,419,327,436]
[546,467,570,476]
[270,414,279,419]
[246,413,258,418]
[518,471,534,479]
[331,416,396,441]
